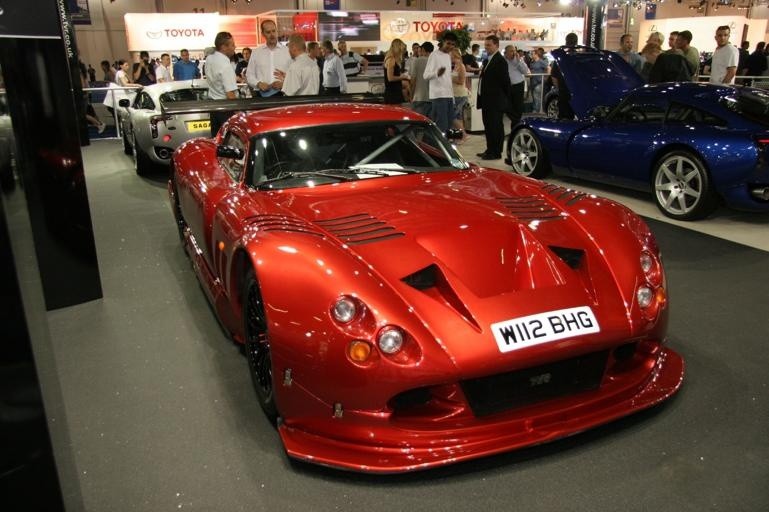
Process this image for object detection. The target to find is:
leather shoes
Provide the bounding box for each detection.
[477,151,501,160]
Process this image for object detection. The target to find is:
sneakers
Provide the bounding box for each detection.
[98,122,106,134]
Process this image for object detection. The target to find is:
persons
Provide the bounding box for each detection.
[551,34,578,119]
[476,35,511,160]
[246,19,293,98]
[367,49,371,55]
[493,28,550,40]
[114,49,199,87]
[463,43,551,126]
[735,41,768,86]
[423,31,470,144]
[230,48,252,82]
[338,40,369,77]
[78,50,117,136]
[384,39,410,104]
[273,35,347,98]
[405,41,435,117]
[206,31,240,99]
[613,25,739,84]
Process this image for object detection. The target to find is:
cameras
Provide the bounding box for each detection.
[144,59,148,64]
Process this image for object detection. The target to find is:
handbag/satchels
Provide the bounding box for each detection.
[102,73,137,111]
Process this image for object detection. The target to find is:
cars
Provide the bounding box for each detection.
[191,60,206,73]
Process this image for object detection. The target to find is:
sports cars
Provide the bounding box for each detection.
[506,42,769,222]
[117,76,249,176]
[160,94,684,478]
